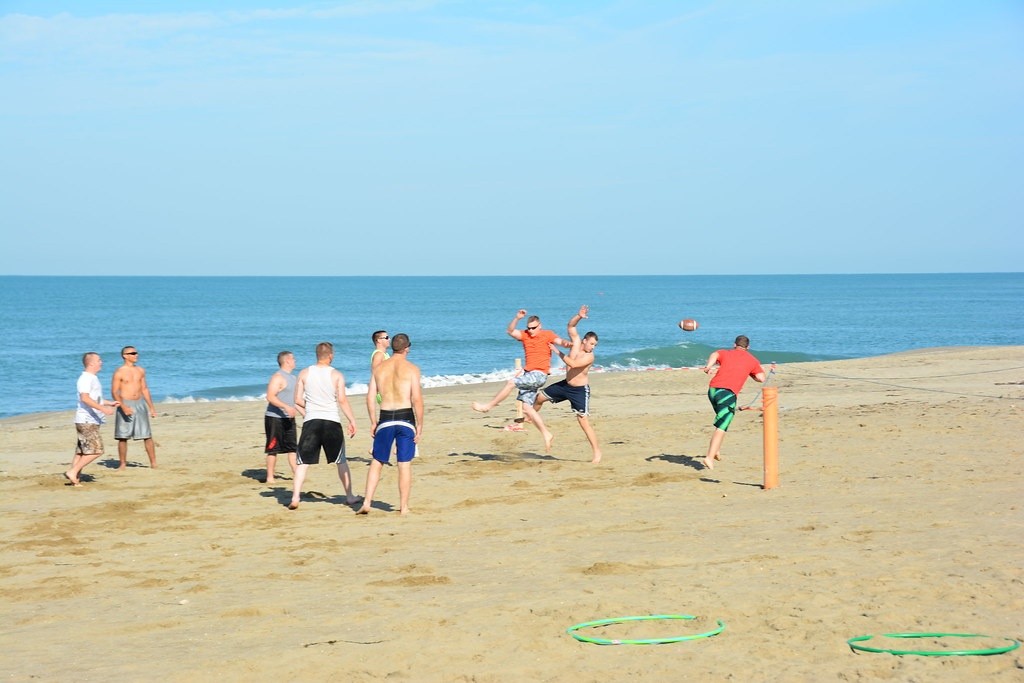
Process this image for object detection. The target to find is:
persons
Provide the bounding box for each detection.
[265,351,306,484]
[354,333,424,515]
[472,309,573,452]
[702,335,767,470]
[514,304,603,464]
[64,352,123,486]
[111,346,157,470]
[288,341,363,510]
[371,330,392,404]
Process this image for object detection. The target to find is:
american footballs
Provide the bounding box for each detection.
[678,319,699,331]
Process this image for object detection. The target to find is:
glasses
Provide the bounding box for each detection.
[124,352,138,355]
[376,336,388,341]
[528,325,539,330]
